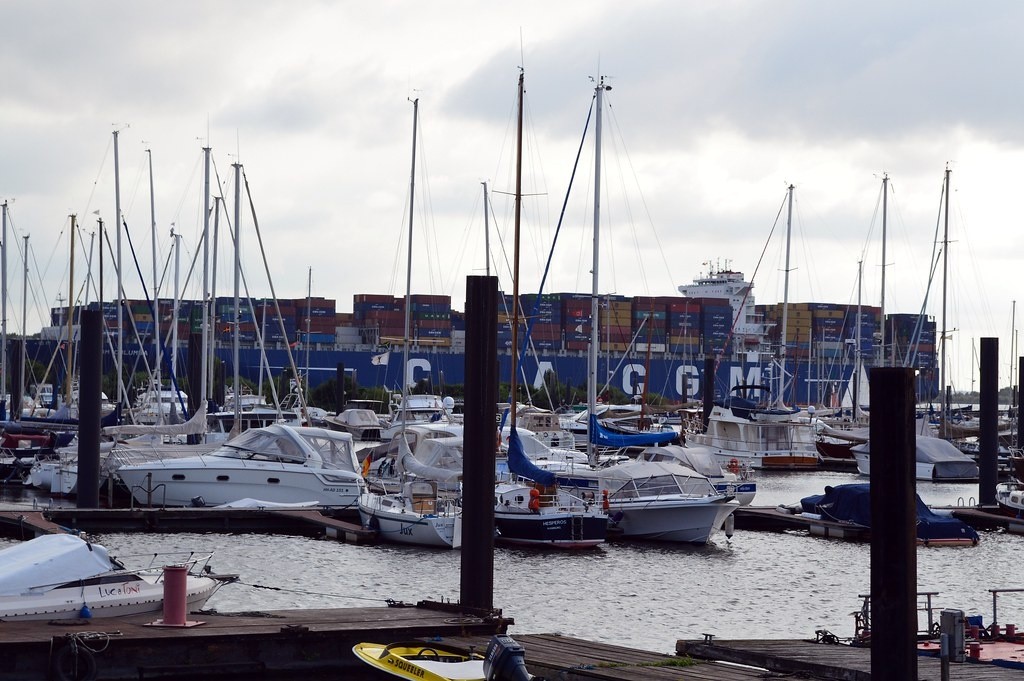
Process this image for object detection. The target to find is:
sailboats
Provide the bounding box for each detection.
[0,67,1024,549]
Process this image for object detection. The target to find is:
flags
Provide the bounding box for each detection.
[376,341,391,350]
[361,450,373,478]
[371,352,389,366]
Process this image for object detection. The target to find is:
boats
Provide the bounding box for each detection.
[0,532,240,627]
[347,630,540,681]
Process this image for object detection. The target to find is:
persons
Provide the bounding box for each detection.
[430,412,442,423]
